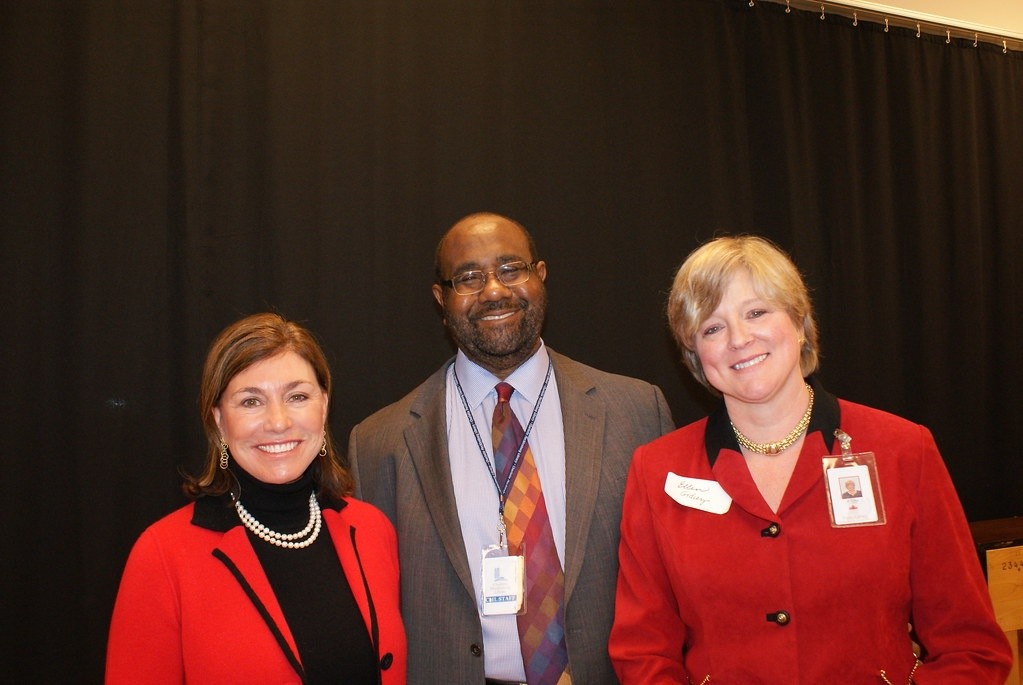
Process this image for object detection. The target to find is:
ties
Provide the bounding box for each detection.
[492,382,572,685]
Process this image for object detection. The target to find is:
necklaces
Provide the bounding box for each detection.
[730,382,814,456]
[230,491,322,548]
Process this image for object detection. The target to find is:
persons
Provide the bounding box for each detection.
[842,480,862,499]
[607,235,1014,685]
[350,211,678,685]
[104,314,407,685]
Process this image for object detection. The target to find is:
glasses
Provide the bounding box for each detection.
[442,262,536,295]
